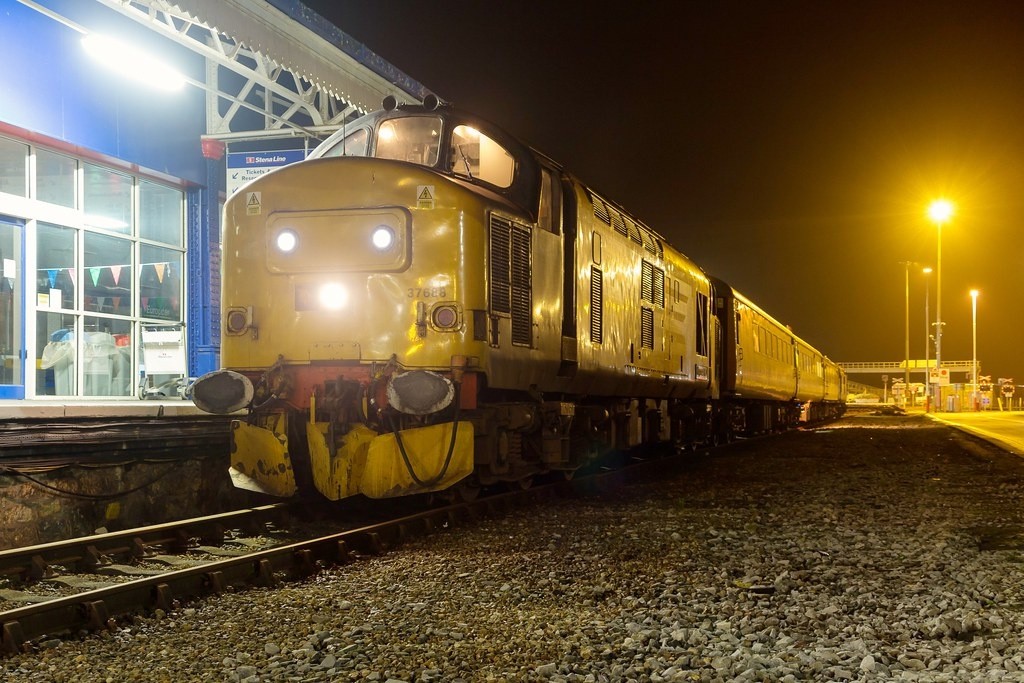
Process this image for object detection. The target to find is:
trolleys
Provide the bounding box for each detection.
[138,321,199,401]
[66,324,131,396]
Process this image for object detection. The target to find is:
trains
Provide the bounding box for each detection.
[184,92,849,517]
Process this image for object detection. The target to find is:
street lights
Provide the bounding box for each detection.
[900,261,917,388]
[970,289,979,394]
[923,267,934,396]
[928,200,952,365]
